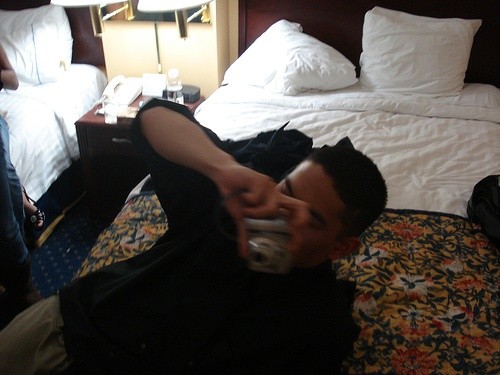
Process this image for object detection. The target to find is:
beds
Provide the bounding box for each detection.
[0,0,105,247]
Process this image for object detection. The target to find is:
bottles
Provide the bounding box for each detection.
[166,68,184,105]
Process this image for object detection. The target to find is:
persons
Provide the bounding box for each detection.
[0,97,388,374]
[0,42,45,307]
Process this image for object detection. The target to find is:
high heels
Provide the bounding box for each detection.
[21,185,45,232]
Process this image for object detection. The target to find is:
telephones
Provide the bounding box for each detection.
[101,77,142,107]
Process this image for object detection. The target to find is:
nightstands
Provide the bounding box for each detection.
[79,85,205,224]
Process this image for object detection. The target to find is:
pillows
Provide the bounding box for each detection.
[223,19,360,95]
[74,0,500,375]
[0,5,75,85]
[360,5,483,99]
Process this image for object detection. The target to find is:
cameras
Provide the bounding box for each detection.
[245,217,291,275]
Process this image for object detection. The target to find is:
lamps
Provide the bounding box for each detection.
[50,0,136,39]
[137,0,211,43]
